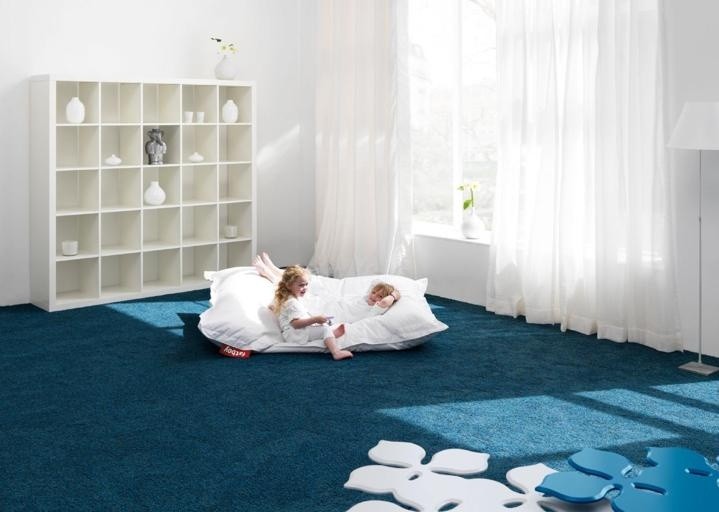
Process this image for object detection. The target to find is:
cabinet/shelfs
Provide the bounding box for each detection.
[29,72,256,314]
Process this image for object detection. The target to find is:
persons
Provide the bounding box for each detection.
[270,263,355,362]
[253,250,401,326]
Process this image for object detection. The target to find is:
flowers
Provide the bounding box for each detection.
[210,37,240,55]
[456,180,485,209]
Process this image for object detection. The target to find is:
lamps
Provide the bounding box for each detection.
[667,99,719,376]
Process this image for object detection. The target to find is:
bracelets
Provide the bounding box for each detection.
[389,293,396,301]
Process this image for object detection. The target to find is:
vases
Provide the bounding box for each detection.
[145,128,167,166]
[221,99,239,123]
[67,96,86,124]
[143,180,166,206]
[215,55,237,79]
[462,207,483,239]
